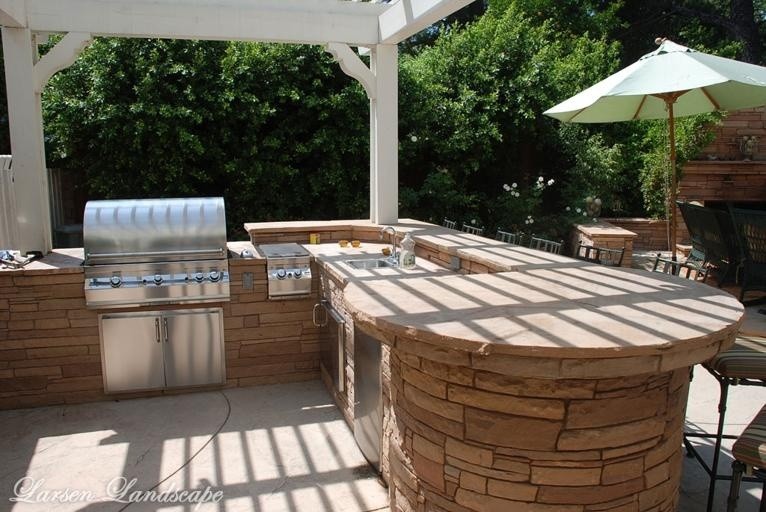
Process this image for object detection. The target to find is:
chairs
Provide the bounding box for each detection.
[442,196,766,511]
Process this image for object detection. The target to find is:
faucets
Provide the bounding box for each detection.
[379,227,395,257]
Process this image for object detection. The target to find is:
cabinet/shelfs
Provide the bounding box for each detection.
[96,306,232,402]
[313,297,345,424]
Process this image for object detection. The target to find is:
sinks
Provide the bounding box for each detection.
[343,256,399,270]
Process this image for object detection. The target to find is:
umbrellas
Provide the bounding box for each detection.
[539,35,766,275]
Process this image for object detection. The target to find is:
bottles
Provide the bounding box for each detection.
[400,232,416,270]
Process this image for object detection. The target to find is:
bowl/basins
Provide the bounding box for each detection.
[350,240,360,247]
[381,248,393,256]
[338,240,349,247]
